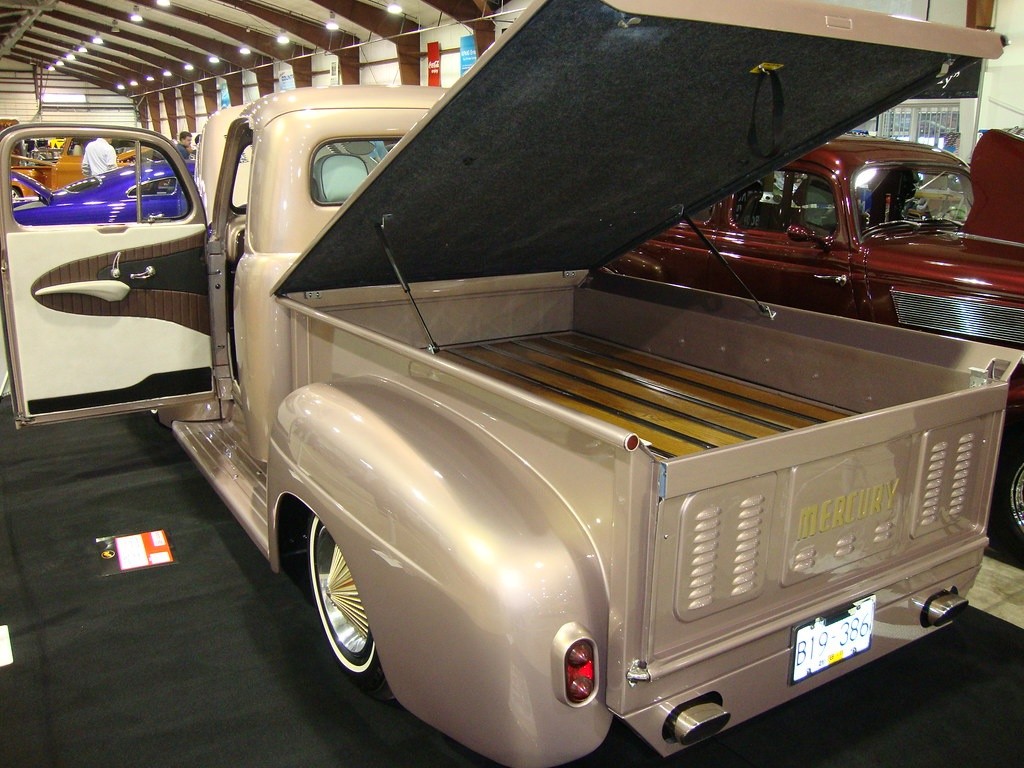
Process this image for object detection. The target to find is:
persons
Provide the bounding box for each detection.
[81,137,118,178]
[176,131,193,160]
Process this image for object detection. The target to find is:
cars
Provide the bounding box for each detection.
[597,129,1023,572]
[0,118,196,225]
[0,0,1024,767]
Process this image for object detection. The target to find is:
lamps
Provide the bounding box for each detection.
[131,6,142,22]
[93,30,103,44]
[117,28,289,90]
[47,41,87,71]
[326,12,339,30]
[111,18,120,32]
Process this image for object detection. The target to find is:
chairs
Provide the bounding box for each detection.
[313,153,368,205]
